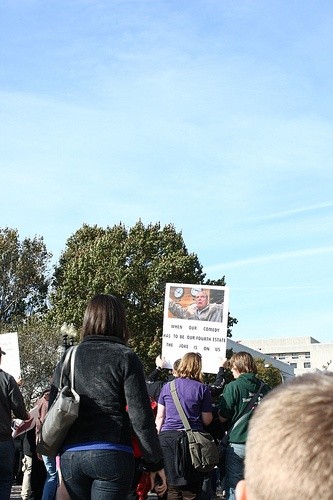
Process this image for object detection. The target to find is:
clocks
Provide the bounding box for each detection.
[190,288,203,296]
[173,287,184,298]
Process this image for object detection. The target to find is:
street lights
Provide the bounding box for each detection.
[60,322,77,352]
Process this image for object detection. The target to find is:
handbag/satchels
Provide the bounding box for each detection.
[218,434,228,457]
[33,345,81,456]
[187,431,219,472]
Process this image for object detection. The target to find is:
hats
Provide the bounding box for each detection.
[0,347,6,355]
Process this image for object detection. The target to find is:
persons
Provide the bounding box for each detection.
[169,291,223,323]
[47,294,167,500]
[218,351,270,500]
[231,372,333,500]
[13,355,234,499]
[0,348,31,500]
